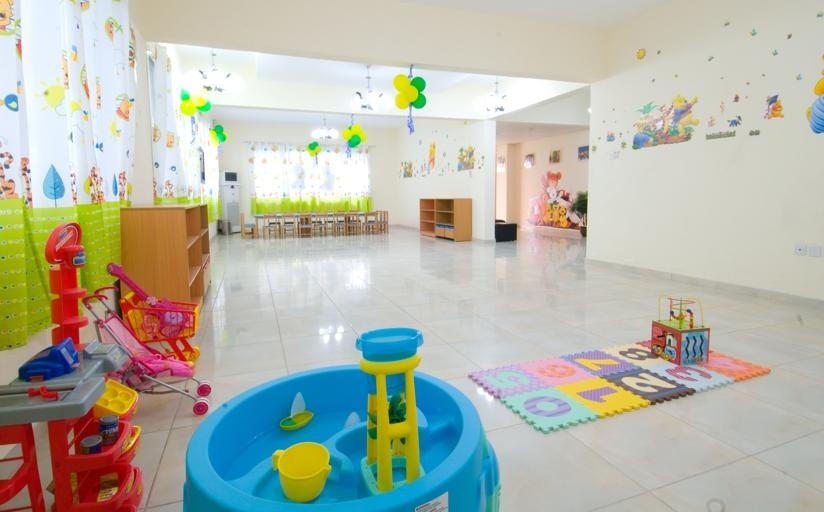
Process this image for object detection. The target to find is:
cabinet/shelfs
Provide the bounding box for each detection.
[419,197,472,241]
[120,203,212,317]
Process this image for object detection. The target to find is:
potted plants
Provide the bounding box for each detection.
[569,191,587,237]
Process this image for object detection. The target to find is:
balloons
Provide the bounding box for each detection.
[180,88,212,116]
[209,124,229,144]
[305,141,322,157]
[342,123,368,147]
[391,74,426,110]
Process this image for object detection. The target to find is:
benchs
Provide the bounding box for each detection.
[495,220,517,243]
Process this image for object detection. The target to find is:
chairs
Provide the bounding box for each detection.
[239,210,389,241]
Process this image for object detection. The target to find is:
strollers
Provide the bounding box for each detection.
[80,285,212,416]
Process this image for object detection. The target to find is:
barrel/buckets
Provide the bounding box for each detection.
[355,329,425,397]
[273,442,332,503]
